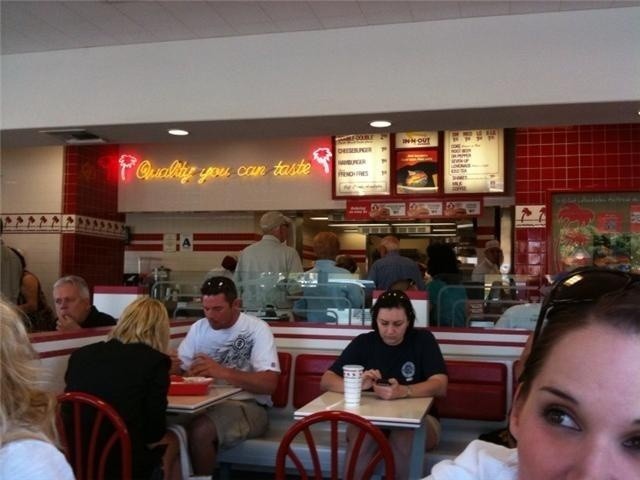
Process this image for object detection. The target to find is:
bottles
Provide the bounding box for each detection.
[164,283,181,319]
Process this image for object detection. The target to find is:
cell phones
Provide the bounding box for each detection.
[376,379,390,386]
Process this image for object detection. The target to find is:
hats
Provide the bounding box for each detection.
[258,211,292,231]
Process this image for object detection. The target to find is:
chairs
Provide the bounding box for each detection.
[49,392,134,480]
[272,410,395,479]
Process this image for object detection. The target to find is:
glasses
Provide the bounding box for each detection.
[520,267,639,380]
[201,279,232,291]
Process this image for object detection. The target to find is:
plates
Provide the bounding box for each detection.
[529,255,539,276]
[397,184,439,192]
[595,259,629,268]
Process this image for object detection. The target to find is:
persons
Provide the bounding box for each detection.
[320,289,449,480]
[418,267,640,480]
[0,292,77,480]
[60,296,195,480]
[0,215,119,331]
[169,276,282,479]
[471,240,504,303]
[231,211,469,327]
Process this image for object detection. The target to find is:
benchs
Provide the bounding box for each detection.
[208,348,525,480]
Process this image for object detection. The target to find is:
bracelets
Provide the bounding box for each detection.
[401,385,413,398]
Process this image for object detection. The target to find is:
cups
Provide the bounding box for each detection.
[343,364,363,404]
